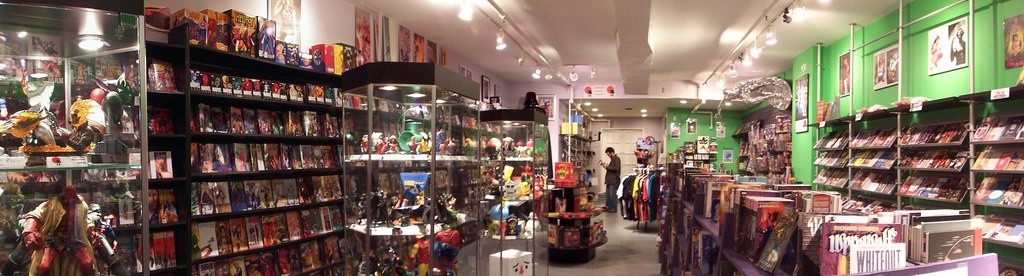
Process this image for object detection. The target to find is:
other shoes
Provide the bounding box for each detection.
[600,209,609,212]
[608,209,617,213]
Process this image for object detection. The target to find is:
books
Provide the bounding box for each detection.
[95,51,178,272]
[813,125,907,215]
[970,115,1024,276]
[683,165,982,276]
[190,68,403,276]
[900,120,970,210]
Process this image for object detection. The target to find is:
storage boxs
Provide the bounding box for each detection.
[144,5,362,78]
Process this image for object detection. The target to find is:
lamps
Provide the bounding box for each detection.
[782,8,792,23]
[495,29,506,51]
[766,26,777,46]
[570,66,578,81]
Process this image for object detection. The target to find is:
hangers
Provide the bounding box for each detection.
[629,168,660,177]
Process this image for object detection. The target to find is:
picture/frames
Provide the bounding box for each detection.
[928,16,968,76]
[873,44,899,90]
[838,50,850,98]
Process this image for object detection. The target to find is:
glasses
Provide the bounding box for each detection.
[607,153,610,155]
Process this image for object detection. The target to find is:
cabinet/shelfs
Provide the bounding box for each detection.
[0,21,608,276]
[657,85,1024,276]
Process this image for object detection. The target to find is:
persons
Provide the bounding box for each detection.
[22,185,118,276]
[357,184,457,227]
[601,147,621,213]
[359,129,455,155]
[357,224,460,276]
[484,166,541,197]
